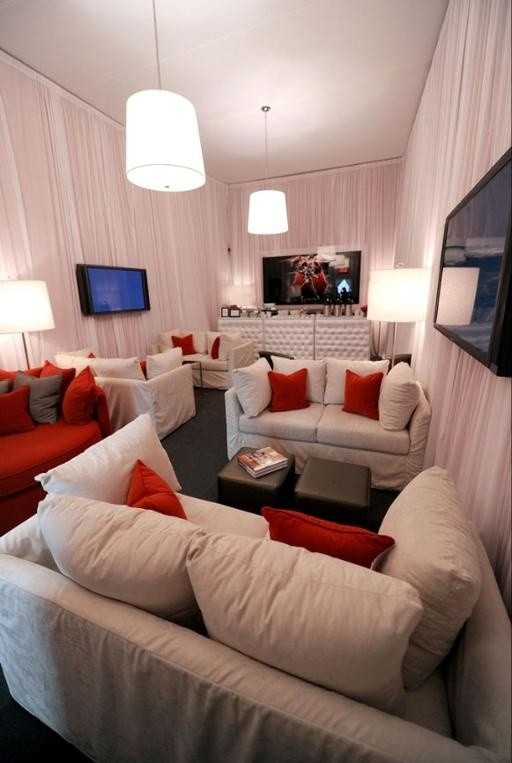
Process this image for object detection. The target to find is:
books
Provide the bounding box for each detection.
[235,460,288,478]
[238,446,289,472]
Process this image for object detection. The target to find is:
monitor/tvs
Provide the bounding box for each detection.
[76,264,151,316]
[432,146,512,378]
[262,250,362,314]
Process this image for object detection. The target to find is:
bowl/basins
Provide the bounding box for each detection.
[263,301,277,309]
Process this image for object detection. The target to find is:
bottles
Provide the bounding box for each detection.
[322,290,353,316]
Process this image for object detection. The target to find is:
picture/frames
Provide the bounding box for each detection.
[228,306,241,317]
[220,305,229,318]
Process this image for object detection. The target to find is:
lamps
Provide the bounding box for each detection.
[364,258,433,372]
[243,104,290,236]
[434,264,481,328]
[0,278,59,370]
[119,0,207,195]
